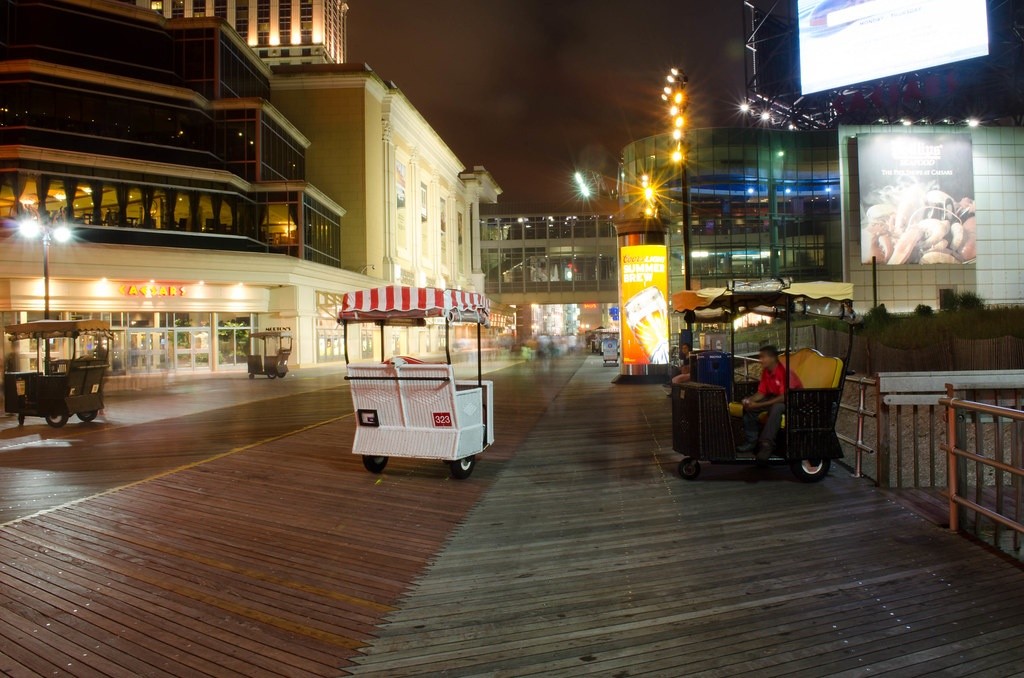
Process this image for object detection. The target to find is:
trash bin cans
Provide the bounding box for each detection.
[688,349,731,402]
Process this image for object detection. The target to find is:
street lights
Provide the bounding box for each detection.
[17,204,74,374]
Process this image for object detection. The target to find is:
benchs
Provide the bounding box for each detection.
[729,347,844,432]
[34,359,107,400]
[347,362,486,461]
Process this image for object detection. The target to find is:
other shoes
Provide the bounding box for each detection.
[756,447,773,460]
[736,443,754,453]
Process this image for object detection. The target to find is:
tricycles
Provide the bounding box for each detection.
[337,284,494,476]
[4,319,111,426]
[246,332,293,377]
[671,279,853,484]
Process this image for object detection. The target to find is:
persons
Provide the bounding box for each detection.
[735,344,804,464]
[672,343,693,384]
